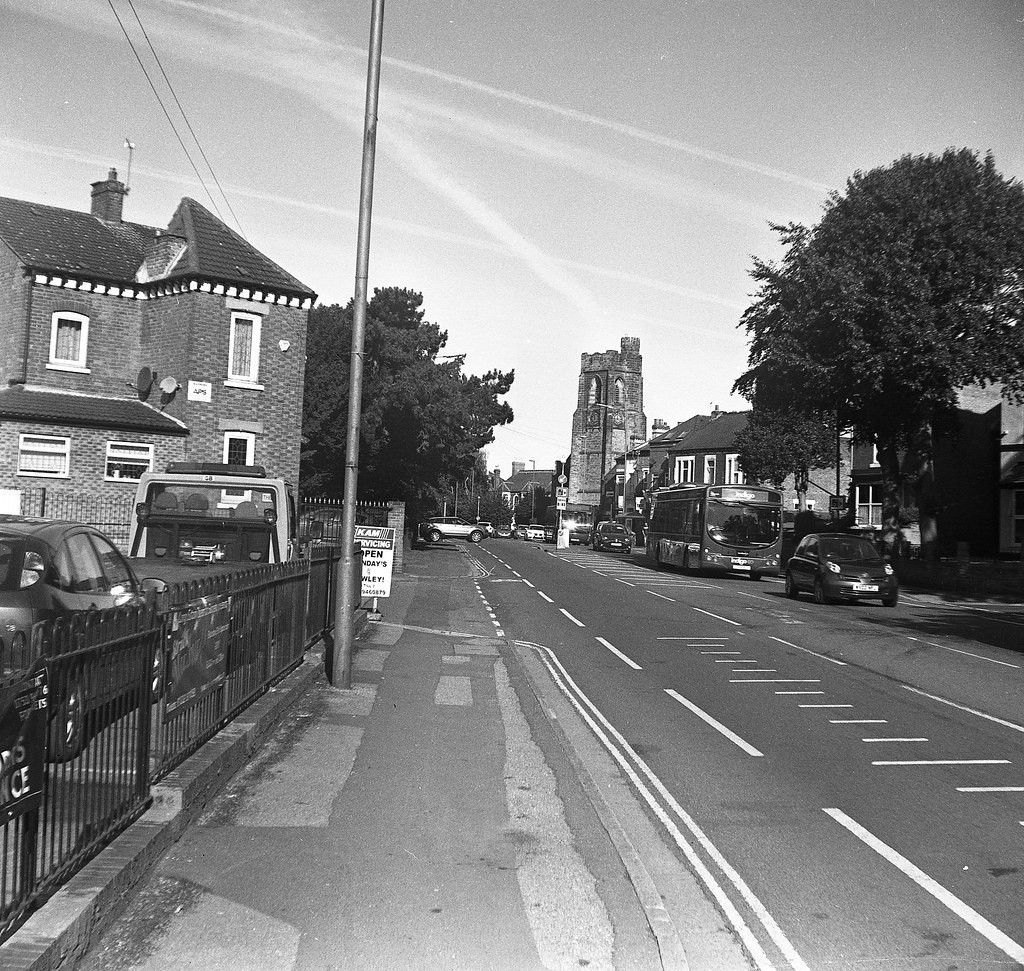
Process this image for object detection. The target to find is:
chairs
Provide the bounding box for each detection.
[232,501,258,519]
[153,492,177,514]
[184,493,212,517]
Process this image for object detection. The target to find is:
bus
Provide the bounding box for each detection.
[645,483,783,580]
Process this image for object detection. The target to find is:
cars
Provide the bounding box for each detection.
[514,524,528,539]
[525,524,546,542]
[544,527,558,542]
[565,524,591,545]
[593,522,631,554]
[495,526,511,538]
[785,532,899,607]
[0,513,168,765]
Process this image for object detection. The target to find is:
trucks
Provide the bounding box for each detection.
[97,463,297,662]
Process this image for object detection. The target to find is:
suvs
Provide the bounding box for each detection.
[299,508,373,540]
[477,521,495,538]
[419,516,488,542]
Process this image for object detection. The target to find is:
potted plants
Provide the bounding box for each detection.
[111,463,123,478]
